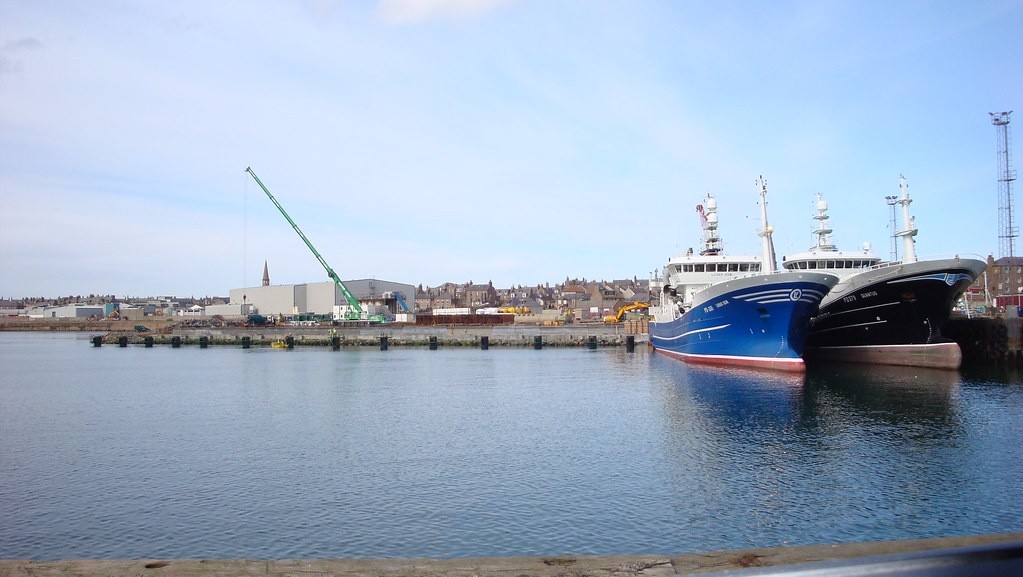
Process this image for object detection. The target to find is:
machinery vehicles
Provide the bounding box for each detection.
[245,167,386,327]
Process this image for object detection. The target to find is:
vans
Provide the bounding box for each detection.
[244,315,267,327]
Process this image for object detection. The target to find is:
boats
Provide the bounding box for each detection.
[648,174,841,370]
[271,340,289,349]
[780,173,987,370]
